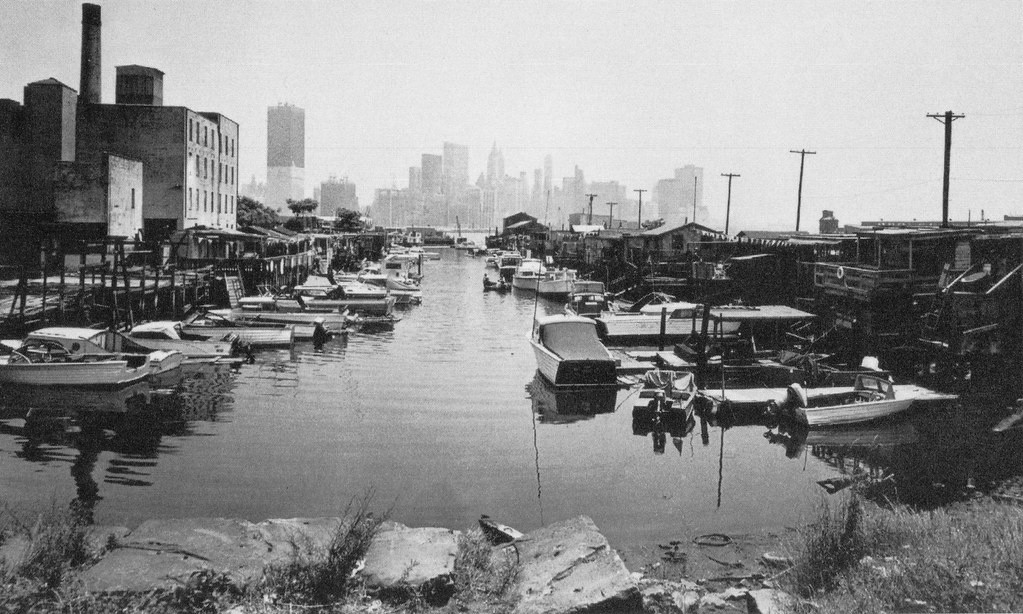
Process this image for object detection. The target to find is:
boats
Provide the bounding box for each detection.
[1,224,440,384]
[525,370,615,500]
[1,384,188,514]
[450,236,926,424]
[631,420,693,455]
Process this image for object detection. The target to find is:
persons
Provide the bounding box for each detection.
[483,273,505,288]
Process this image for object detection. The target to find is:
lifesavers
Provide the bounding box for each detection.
[836,267,844,279]
